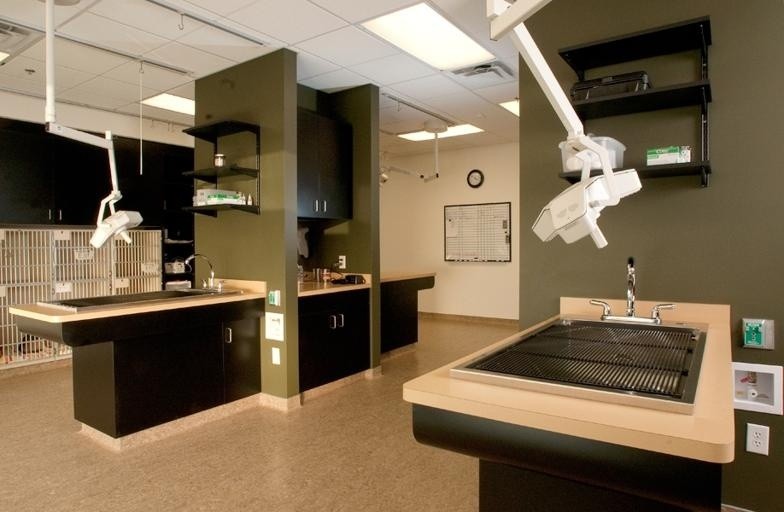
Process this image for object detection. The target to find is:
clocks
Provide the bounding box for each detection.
[467,170,483,188]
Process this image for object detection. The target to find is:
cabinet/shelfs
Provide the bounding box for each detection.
[297,289,370,394]
[218,317,261,402]
[295,106,354,221]
[556,13,711,188]
[0,114,195,228]
[178,119,261,217]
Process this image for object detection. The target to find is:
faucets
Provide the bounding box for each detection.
[626,257,635,315]
[184,254,215,289]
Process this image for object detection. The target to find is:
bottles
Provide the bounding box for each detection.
[192,192,209,206]
[238,190,253,206]
[679,145,691,163]
[297,265,305,283]
[298,281,304,282]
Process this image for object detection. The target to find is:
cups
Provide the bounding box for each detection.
[313,282,330,290]
[311,267,331,283]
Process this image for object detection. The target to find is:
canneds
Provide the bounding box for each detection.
[212,153,226,169]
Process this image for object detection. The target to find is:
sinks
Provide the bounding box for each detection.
[36,290,241,314]
[450,316,710,416]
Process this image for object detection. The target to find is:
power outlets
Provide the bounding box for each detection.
[742,317,774,350]
[744,422,770,455]
